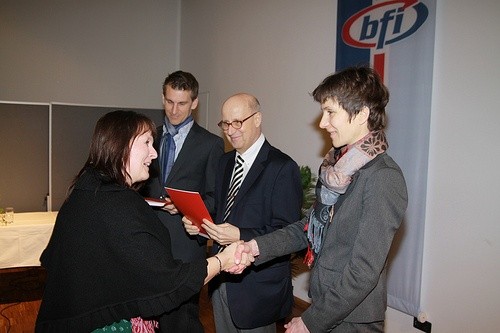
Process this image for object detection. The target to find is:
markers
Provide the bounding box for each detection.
[46,194,50,212]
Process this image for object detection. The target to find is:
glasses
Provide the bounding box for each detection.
[218,113,257,130]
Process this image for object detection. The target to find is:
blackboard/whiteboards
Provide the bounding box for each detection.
[51,102,166,212]
[0,100,51,214]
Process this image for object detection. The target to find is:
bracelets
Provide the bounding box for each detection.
[214,256,222,275]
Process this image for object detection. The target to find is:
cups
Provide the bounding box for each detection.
[5,207,14,225]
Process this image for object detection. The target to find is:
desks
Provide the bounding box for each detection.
[0,209,59,274]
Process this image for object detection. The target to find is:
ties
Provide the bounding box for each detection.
[218,155,244,255]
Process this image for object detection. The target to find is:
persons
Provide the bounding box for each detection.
[135,71,226,264]
[182,92,305,333]
[35,110,255,333]
[223,66,408,333]
